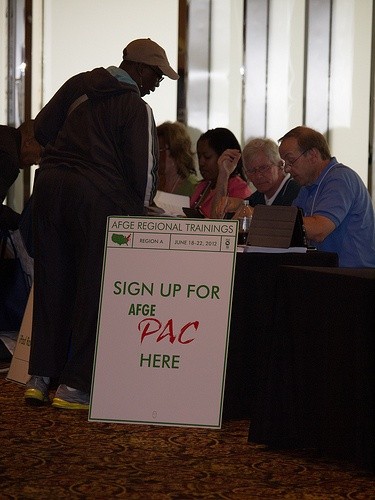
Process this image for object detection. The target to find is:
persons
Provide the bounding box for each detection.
[0,120,44,373]
[23,39,165,411]
[236,126,375,270]
[153,122,201,199]
[190,128,255,219]
[213,138,298,246]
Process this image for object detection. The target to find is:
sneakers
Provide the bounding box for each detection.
[52,384,90,410]
[23,375,52,402]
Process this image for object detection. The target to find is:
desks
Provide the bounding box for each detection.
[247,267,375,481]
[223,233,339,420]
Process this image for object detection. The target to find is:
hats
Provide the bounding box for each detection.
[124,38,182,80]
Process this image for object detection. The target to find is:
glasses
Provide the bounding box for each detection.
[245,162,277,177]
[279,147,311,172]
[143,64,164,86]
[159,146,171,156]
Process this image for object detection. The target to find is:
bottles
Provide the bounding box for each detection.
[237,200,253,245]
